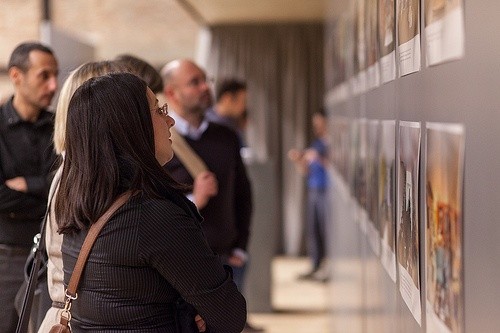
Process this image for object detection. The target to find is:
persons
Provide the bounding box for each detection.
[54,70,250,333]
[37,56,155,332]
[1,40,70,332]
[211,74,252,128]
[156,56,257,315]
[288,107,346,284]
[106,51,165,98]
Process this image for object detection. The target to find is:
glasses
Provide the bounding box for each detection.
[150,103,168,115]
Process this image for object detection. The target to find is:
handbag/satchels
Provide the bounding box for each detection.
[13,245,52,331]
